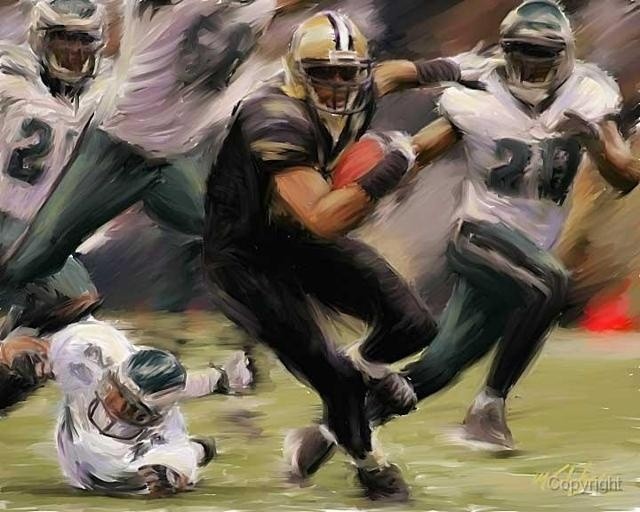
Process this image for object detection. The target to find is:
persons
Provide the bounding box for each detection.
[1,1,384,498]
[202,9,508,504]
[280,1,640,486]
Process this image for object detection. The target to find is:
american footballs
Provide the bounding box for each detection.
[333,138,383,193]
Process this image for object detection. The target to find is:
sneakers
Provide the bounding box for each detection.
[292,426,333,478]
[223,350,254,388]
[462,396,513,448]
[193,435,216,464]
[348,349,420,414]
[356,459,410,500]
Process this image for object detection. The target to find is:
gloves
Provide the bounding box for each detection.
[444,42,507,91]
[374,129,416,163]
[553,110,604,147]
[11,352,58,390]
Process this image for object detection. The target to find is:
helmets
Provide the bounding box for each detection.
[287,10,372,118]
[30,2,103,74]
[97,349,186,424]
[499,1,576,87]
